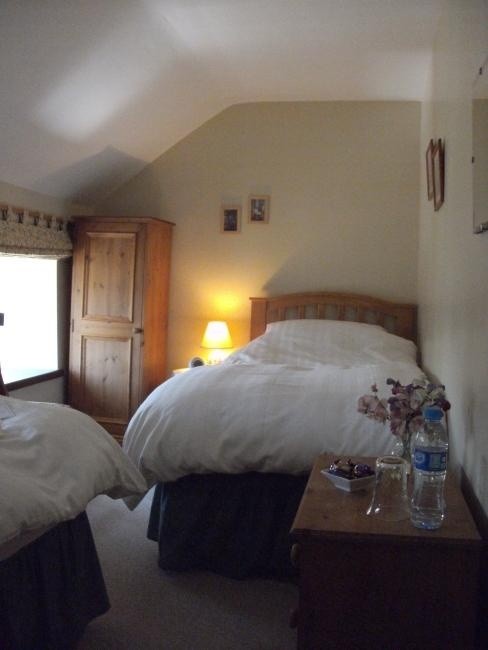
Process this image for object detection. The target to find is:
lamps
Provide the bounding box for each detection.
[200,321,230,366]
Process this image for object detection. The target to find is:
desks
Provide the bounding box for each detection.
[289,457,485,650]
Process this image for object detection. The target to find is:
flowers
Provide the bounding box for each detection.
[357,381,452,447]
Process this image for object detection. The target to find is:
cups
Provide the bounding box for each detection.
[367,456,411,524]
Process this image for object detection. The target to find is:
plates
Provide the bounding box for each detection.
[321,467,374,493]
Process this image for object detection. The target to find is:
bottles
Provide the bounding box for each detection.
[407,404,449,531]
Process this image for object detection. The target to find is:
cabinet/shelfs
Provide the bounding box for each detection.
[66,216,168,440]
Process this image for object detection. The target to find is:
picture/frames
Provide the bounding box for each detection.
[424,135,445,210]
[218,194,270,234]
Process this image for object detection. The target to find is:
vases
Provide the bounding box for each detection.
[388,433,411,478]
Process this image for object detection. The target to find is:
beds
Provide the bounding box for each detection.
[152,297,415,569]
